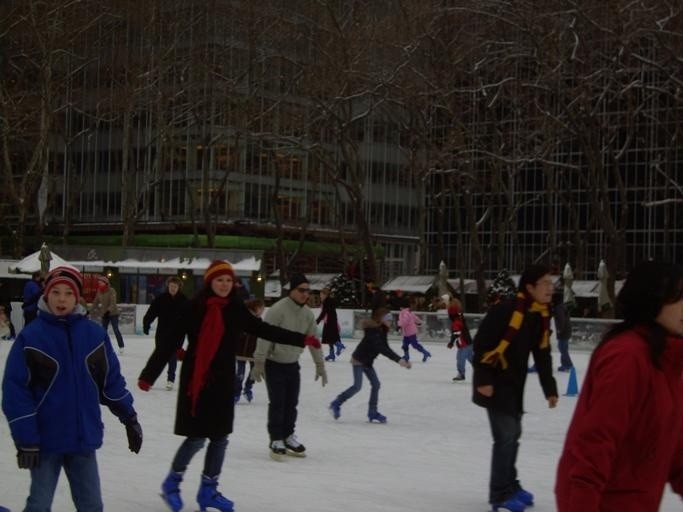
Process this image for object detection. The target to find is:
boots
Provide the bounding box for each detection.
[331,399,340,417]
[368,409,385,421]
[162,467,182,511]
[452,367,465,380]
[325,341,345,361]
[197,475,233,512]
[402,350,430,362]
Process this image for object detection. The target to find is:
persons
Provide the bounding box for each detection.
[236,274,573,423]
[249,275,328,463]
[78,273,204,391]
[1,266,142,512]
[1,271,46,340]
[472,263,558,512]
[137,259,321,512]
[555,258,683,512]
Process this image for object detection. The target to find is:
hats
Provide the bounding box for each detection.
[42,266,83,304]
[399,299,410,308]
[97,276,109,284]
[290,273,309,289]
[204,259,235,287]
[166,277,183,289]
[376,308,393,321]
[448,305,460,315]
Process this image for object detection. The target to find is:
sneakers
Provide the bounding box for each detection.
[118,346,125,353]
[527,360,573,372]
[270,435,305,454]
[166,380,174,390]
[493,488,533,512]
[234,388,252,401]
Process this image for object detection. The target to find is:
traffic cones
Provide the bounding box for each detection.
[563,366,579,396]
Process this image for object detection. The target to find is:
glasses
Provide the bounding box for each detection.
[294,288,310,293]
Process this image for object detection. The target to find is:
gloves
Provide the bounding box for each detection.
[17,446,40,470]
[448,342,453,348]
[138,378,150,391]
[396,323,421,332]
[315,362,328,387]
[87,310,111,319]
[122,413,143,454]
[143,324,150,335]
[249,361,266,382]
[316,317,320,325]
[304,336,320,348]
